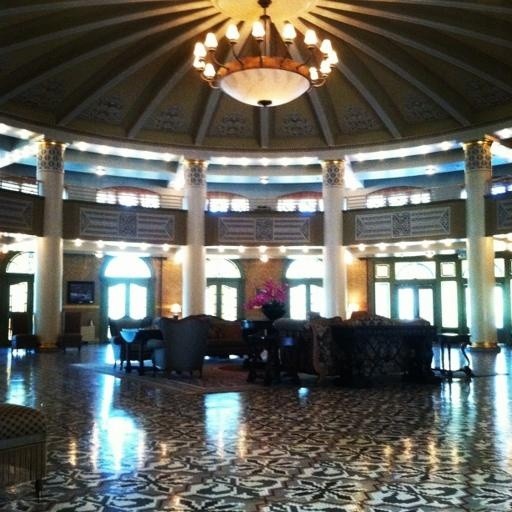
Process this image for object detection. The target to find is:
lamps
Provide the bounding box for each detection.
[191,0,339,109]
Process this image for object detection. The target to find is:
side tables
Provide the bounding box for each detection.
[438,333,471,381]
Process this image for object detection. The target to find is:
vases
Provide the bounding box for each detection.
[261,305,287,320]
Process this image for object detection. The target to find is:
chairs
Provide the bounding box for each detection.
[11,312,33,352]
[63,312,82,354]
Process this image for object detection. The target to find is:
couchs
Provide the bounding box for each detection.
[1,402,47,494]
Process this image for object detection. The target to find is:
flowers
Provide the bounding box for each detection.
[243,279,287,309]
